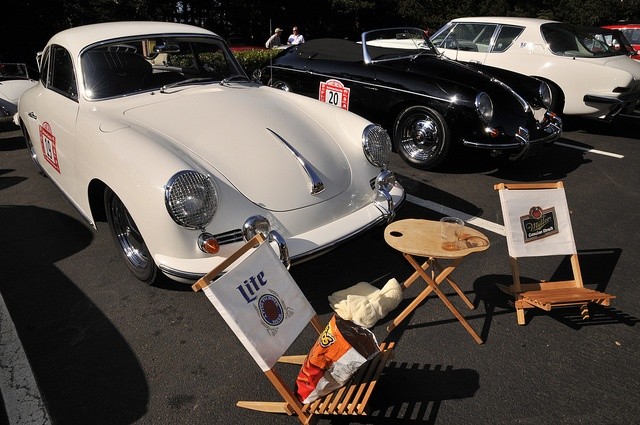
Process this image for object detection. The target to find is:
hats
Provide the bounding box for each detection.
[275,28,283,32]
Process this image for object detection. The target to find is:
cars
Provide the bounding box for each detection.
[262,27,563,170]
[0,62,39,116]
[356,17,640,124]
[13,21,406,285]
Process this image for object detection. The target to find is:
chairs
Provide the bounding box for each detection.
[495,182,616,325]
[192,234,394,425]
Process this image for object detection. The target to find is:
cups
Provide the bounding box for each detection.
[440,217,464,251]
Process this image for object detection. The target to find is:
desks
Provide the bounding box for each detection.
[384,218,490,345]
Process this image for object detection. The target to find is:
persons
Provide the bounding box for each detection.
[265,27,283,48]
[423,26,430,38]
[287,26,305,45]
